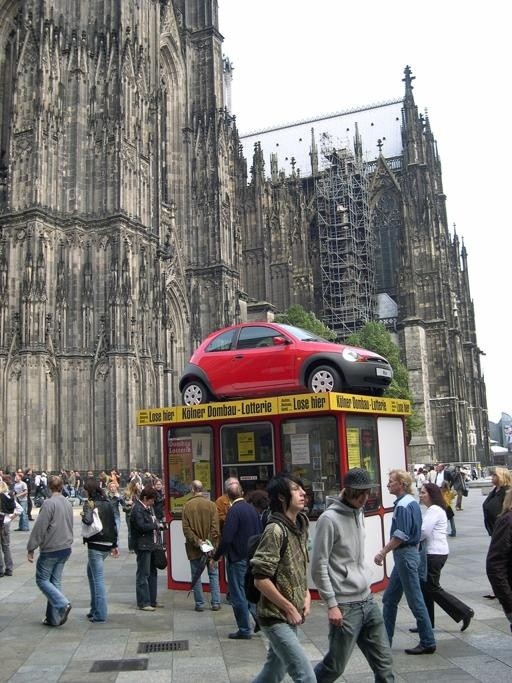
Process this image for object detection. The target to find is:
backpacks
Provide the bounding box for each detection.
[243,520,289,604]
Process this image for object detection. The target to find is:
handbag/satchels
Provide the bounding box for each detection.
[152,523,169,569]
[81,500,102,538]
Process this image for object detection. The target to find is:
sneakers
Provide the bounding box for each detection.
[43,604,107,626]
[253,623,261,634]
[212,604,222,611]
[229,630,251,639]
[142,603,164,611]
[1,518,34,577]
[449,505,465,537]
[195,605,205,612]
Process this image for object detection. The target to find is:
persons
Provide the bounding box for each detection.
[374,468,436,655]
[311,466,398,682]
[244,473,322,682]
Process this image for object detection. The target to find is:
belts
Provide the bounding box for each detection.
[392,543,416,548]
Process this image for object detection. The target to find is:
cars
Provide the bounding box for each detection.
[410,459,483,481]
[177,317,395,408]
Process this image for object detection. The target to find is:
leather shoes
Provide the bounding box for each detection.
[405,642,438,654]
[460,607,474,632]
[408,628,421,632]
[483,592,497,600]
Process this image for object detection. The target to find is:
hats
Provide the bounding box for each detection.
[344,467,380,490]
